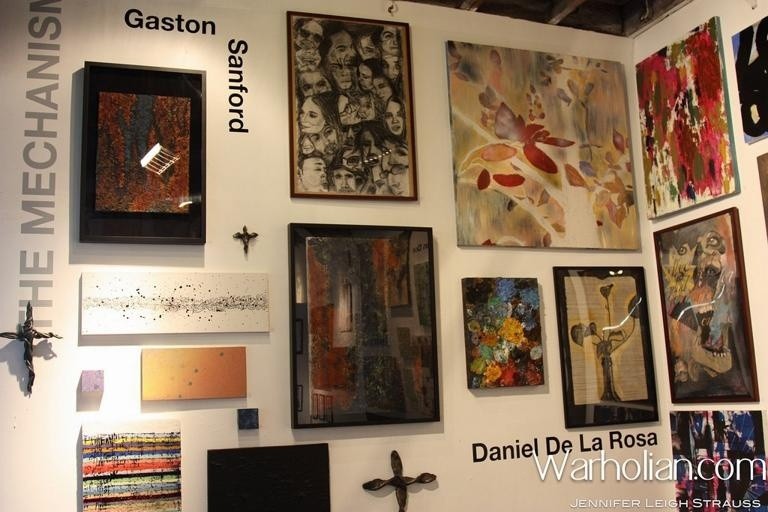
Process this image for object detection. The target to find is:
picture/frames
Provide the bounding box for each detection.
[79,60,207,246]
[653,206,761,404]
[286,11,418,201]
[287,223,440,430]
[553,266,660,429]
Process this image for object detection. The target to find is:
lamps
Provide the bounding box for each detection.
[80,368,103,393]
